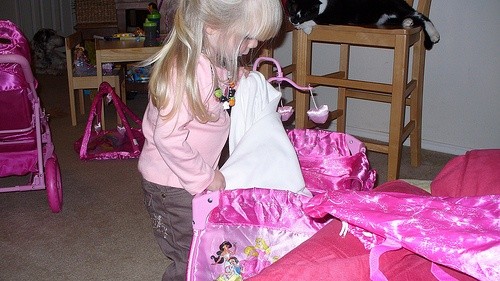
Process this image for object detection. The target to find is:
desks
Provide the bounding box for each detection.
[94,35,162,131]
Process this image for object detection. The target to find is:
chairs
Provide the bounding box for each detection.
[291,0,432,180]
[63,32,127,128]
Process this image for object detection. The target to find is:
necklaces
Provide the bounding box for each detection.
[199,50,237,111]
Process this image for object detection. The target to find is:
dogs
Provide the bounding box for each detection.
[29,27,67,76]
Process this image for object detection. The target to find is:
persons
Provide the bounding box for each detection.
[135,0,282,281]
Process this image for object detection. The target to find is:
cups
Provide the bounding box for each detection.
[142,9,161,47]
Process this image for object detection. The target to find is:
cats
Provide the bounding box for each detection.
[285,0,440,51]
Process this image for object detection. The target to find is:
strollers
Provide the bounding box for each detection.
[0,20,64,214]
